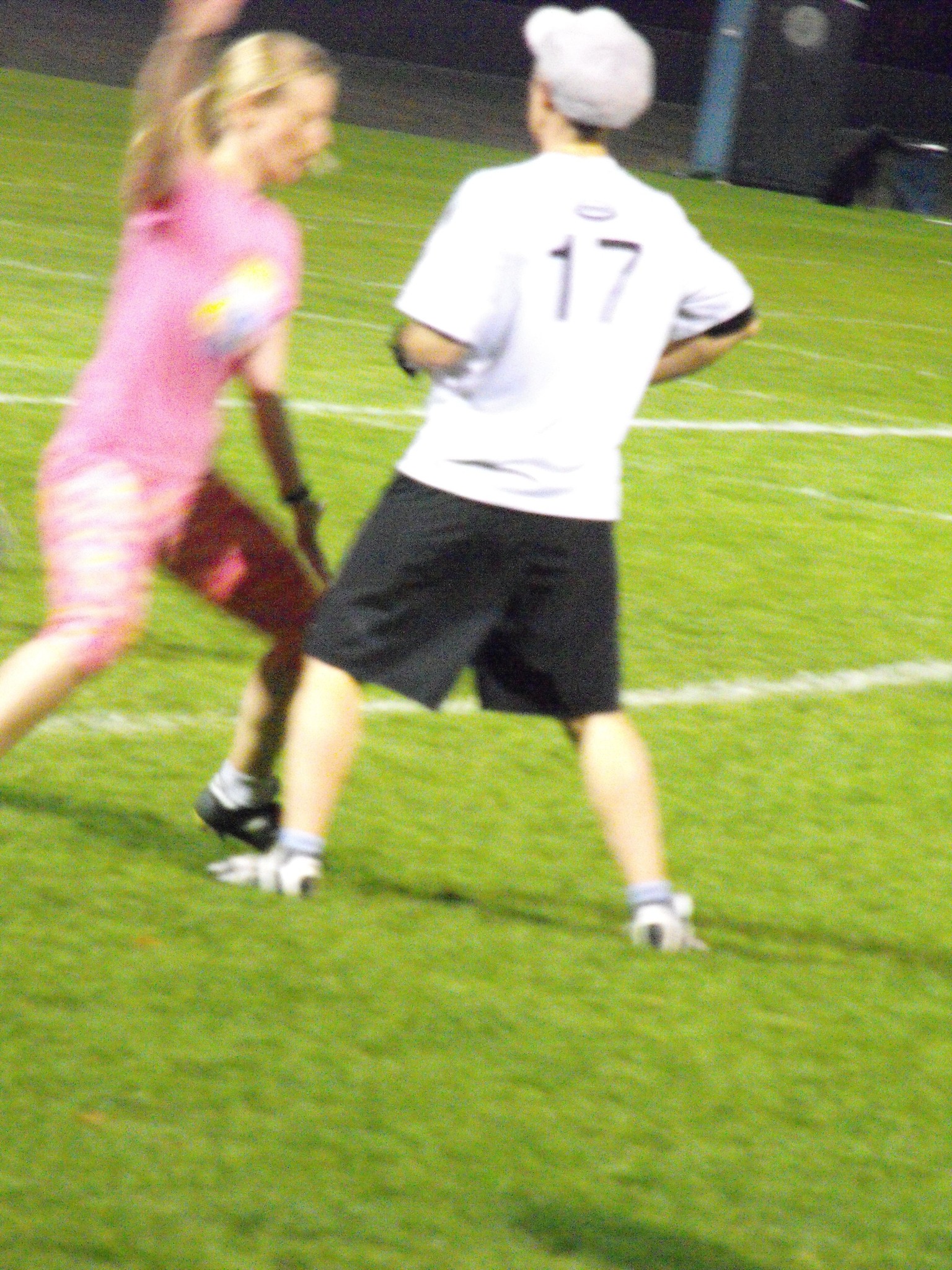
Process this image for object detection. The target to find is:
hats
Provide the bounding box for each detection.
[525,6,657,129]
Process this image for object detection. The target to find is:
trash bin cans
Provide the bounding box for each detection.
[687,0,871,197]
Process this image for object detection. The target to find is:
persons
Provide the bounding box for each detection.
[0,0,343,855]
[206,6,762,960]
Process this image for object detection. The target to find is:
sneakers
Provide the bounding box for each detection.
[187,770,280,854]
[206,851,322,902]
[631,905,709,954]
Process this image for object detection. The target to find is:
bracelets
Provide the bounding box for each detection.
[284,485,310,504]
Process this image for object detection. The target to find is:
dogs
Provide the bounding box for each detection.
[819,127,909,207]
